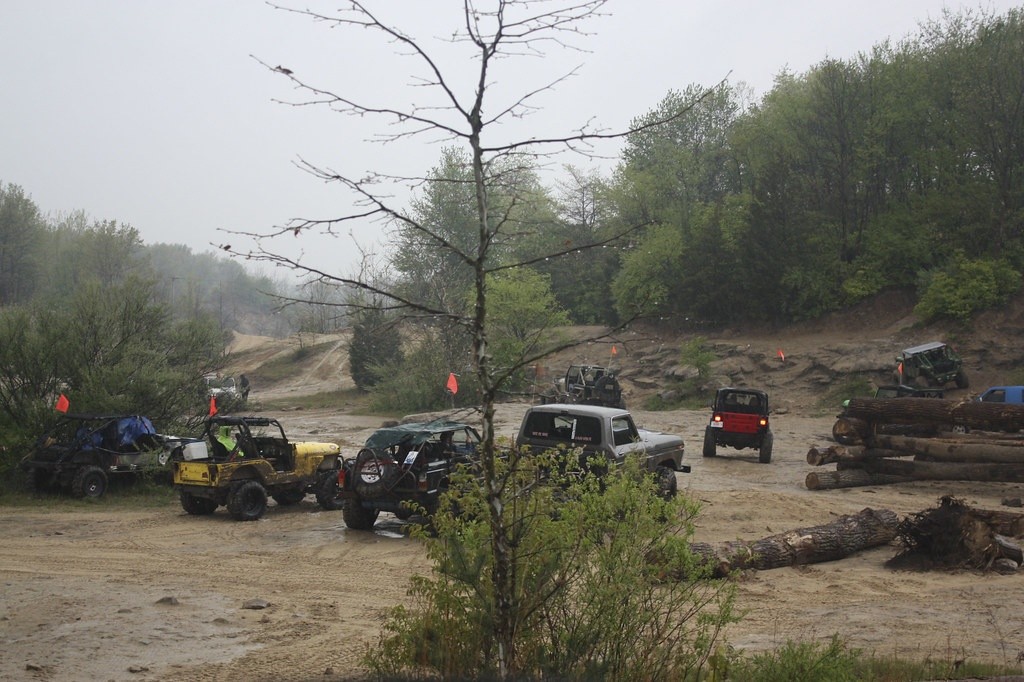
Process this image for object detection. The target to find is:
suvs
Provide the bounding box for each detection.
[893,342,969,395]
[171,416,344,522]
[832,386,968,438]
[701,388,774,463]
[340,423,510,530]
[516,404,693,501]
[540,365,628,411]
[21,413,188,502]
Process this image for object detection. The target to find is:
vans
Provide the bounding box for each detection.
[969,385,1024,404]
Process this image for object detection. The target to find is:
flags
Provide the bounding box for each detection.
[447,372,460,394]
[611,346,617,355]
[55,394,70,414]
[210,398,219,414]
[898,363,903,374]
[778,348,785,361]
[535,365,541,376]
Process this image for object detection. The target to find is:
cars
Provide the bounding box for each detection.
[206,377,237,396]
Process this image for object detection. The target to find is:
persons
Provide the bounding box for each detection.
[239,374,251,401]
[217,426,245,457]
[584,369,593,382]
[431,431,474,462]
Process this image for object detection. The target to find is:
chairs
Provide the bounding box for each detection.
[209,435,242,461]
[100,425,120,453]
[234,434,277,463]
[729,394,738,403]
[748,397,761,407]
[593,370,604,381]
